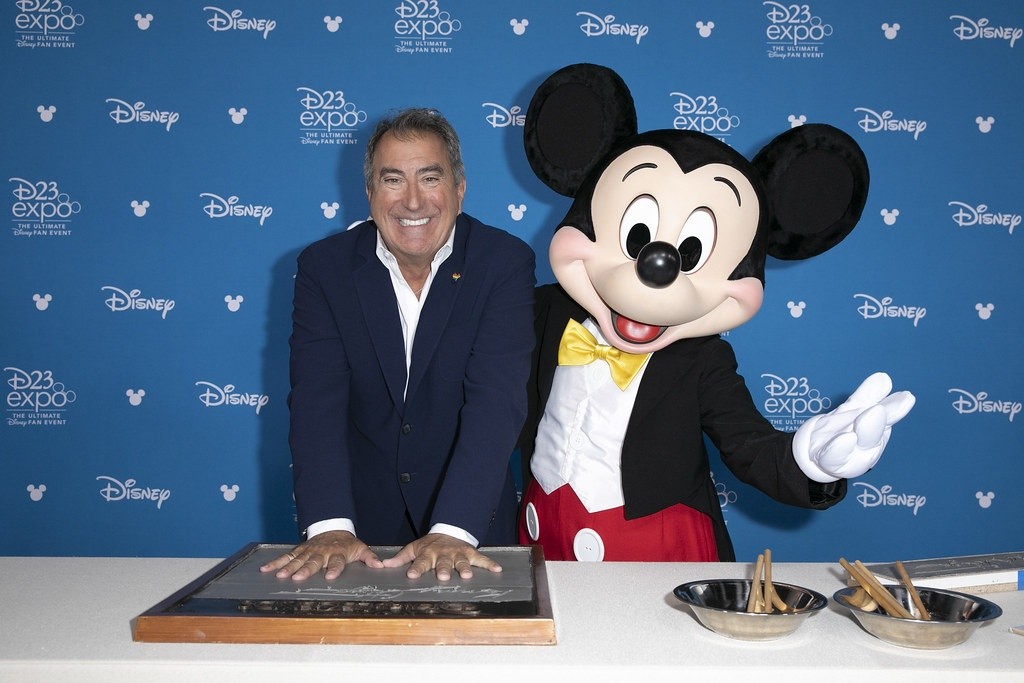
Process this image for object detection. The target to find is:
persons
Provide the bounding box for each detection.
[260,107,538,581]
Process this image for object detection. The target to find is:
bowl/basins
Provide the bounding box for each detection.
[671,578,827,641]
[832,583,998,650]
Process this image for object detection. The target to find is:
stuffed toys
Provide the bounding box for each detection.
[512,63,917,564]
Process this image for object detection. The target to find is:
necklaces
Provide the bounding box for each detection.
[415,289,422,295]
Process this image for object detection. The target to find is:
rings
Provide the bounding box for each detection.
[285,553,294,560]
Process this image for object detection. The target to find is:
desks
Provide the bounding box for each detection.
[0,554,1024,683]
